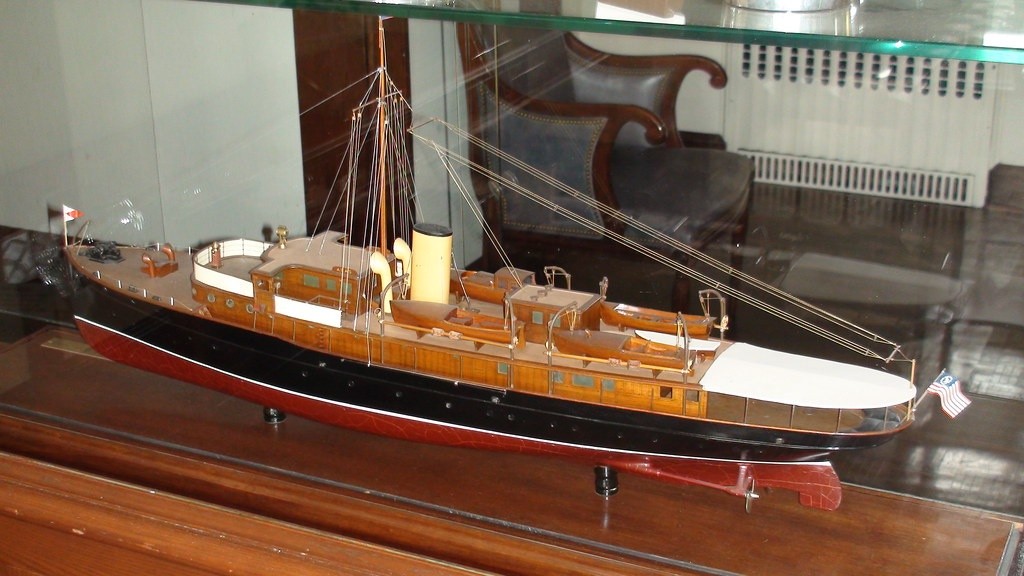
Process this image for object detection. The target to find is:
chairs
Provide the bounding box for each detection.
[456,19,757,328]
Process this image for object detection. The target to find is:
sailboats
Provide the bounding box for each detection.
[60,13,925,507]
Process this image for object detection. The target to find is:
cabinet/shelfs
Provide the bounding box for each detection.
[206,0,1024,69]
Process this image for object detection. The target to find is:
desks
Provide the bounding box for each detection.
[0,325,1023,576]
